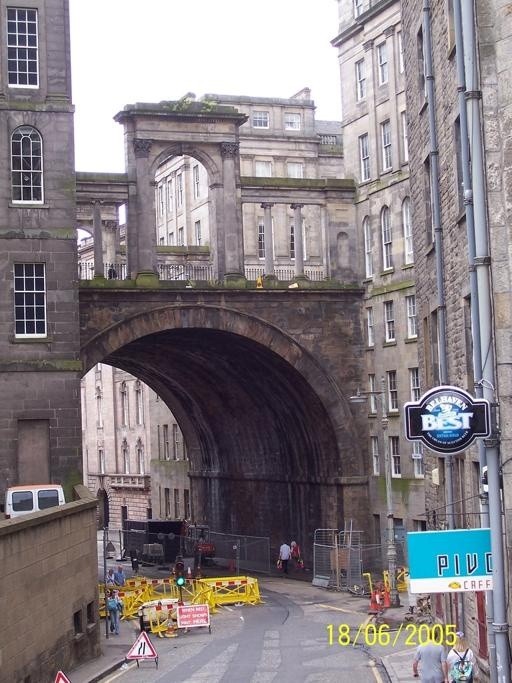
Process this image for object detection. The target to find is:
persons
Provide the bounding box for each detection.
[115,564,126,586]
[413,629,448,682]
[289,540,301,569]
[109,591,120,634]
[107,569,115,583]
[446,631,481,682]
[280,539,292,572]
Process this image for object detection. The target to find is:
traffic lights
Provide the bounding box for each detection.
[175,555,185,584]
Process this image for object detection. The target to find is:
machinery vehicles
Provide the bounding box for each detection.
[178,521,216,567]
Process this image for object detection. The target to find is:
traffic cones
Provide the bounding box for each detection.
[165,611,178,637]
[186,564,193,579]
[228,556,235,571]
[255,271,264,288]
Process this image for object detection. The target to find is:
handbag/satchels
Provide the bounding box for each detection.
[296,558,304,569]
[277,560,282,569]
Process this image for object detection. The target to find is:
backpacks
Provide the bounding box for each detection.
[449,648,473,683]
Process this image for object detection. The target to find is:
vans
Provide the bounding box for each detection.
[4,483,65,518]
